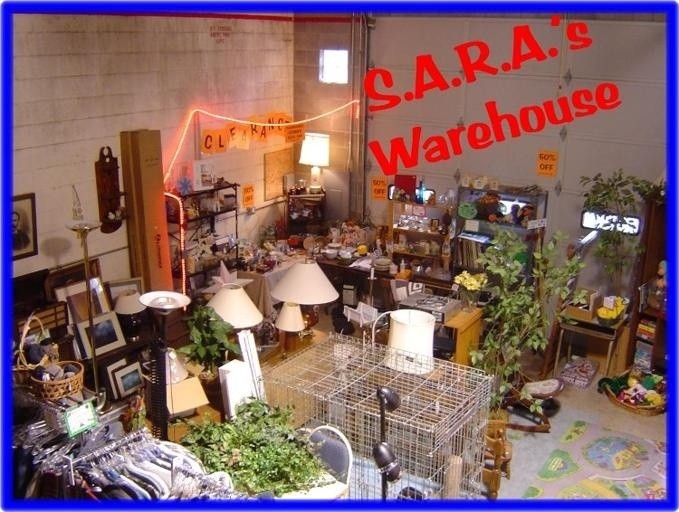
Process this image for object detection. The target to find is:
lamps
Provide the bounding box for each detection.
[298,129,332,176]
[63,185,101,236]
[205,283,264,329]
[269,260,340,305]
[274,303,305,333]
[111,289,147,337]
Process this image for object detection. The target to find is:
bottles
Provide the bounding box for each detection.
[418,181,423,205]
[229,233,234,249]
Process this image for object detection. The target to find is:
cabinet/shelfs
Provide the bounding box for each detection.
[164,181,241,313]
[626,197,668,374]
[452,182,549,301]
[386,183,456,296]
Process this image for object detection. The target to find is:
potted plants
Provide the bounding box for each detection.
[176,304,242,399]
[577,168,666,314]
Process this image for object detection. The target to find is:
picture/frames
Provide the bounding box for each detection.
[8,192,38,261]
[52,275,113,324]
[105,357,129,402]
[111,360,147,401]
[73,310,127,360]
[104,277,145,308]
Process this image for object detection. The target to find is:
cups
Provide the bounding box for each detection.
[306,242,316,264]
[322,249,338,261]
[328,243,342,256]
[277,240,287,255]
